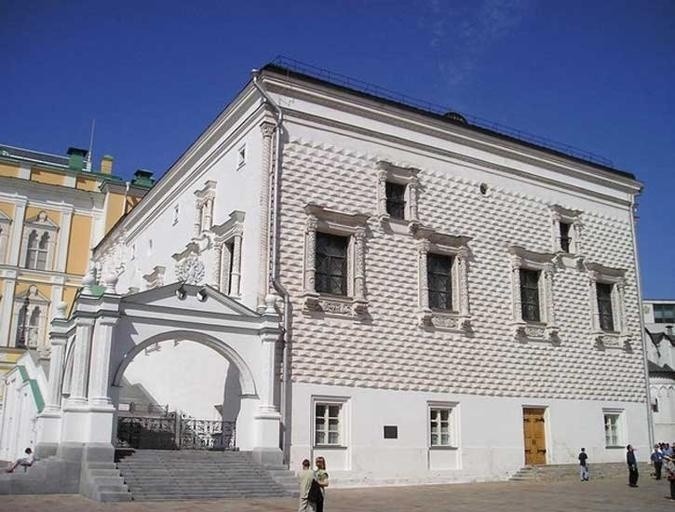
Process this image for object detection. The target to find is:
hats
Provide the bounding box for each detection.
[653,445,659,448]
[670,442,675,448]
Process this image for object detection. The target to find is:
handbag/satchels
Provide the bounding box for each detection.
[667,470,675,480]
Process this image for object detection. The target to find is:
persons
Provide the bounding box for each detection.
[296,459,315,512]
[626,444,640,487]
[5,448,34,473]
[310,456,329,512]
[578,447,588,480]
[650,442,675,501]
